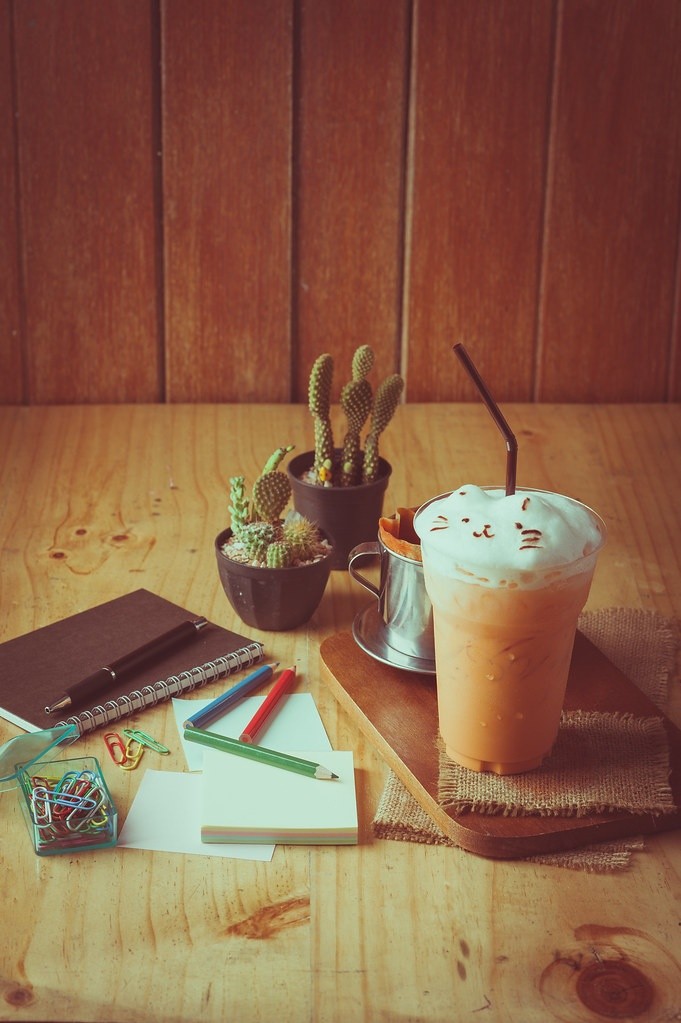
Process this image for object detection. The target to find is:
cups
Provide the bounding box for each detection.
[346,506,435,662]
[414,486,608,769]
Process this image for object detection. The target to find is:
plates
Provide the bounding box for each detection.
[351,599,436,676]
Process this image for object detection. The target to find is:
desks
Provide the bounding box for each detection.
[0,403,681,1023]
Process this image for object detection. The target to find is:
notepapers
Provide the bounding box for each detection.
[198,746,362,847]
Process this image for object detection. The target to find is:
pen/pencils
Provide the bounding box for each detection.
[46,615,209,713]
[183,660,341,781]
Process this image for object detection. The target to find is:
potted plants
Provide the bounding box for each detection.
[286,344,405,571]
[214,444,335,633]
